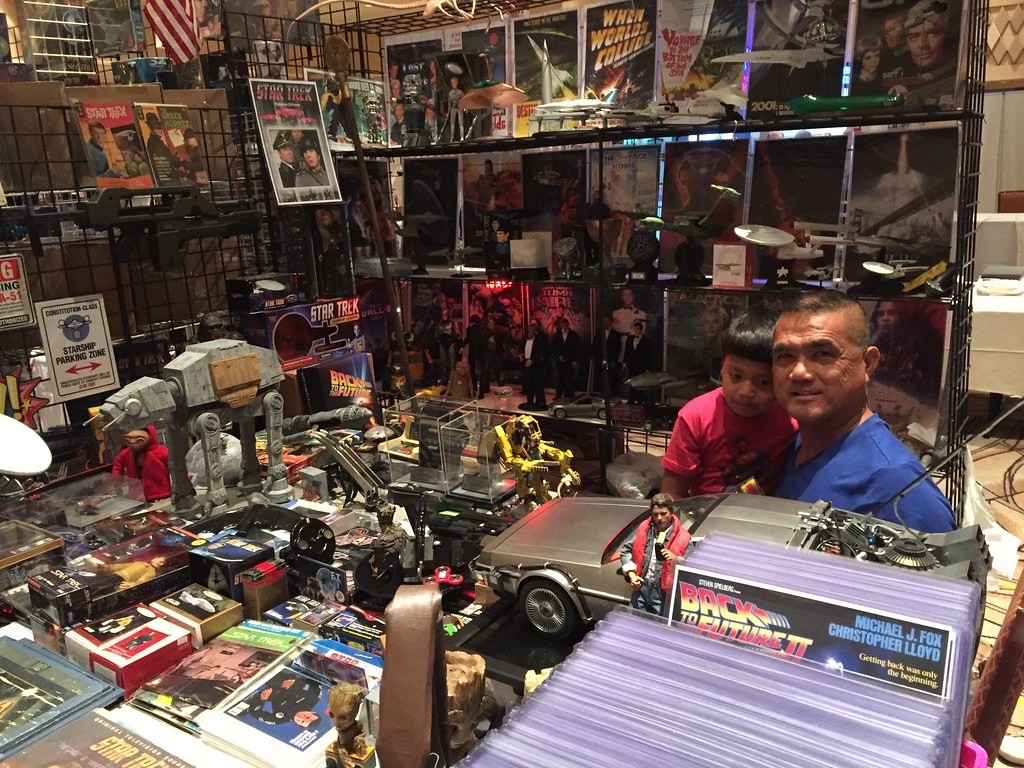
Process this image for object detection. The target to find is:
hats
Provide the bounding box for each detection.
[271,133,293,150]
[144,112,162,126]
[183,128,194,139]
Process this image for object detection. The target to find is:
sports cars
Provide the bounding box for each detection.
[471,494,993,649]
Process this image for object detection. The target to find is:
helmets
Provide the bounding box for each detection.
[299,136,322,156]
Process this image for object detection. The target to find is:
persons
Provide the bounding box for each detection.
[110,426,170,502]
[870,297,922,398]
[849,0,957,102]
[84,555,169,596]
[768,288,957,532]
[681,309,730,383]
[659,308,798,500]
[84,6,656,344]
[670,150,726,217]
[248,671,320,729]
[620,491,691,616]
[420,314,657,411]
[172,648,259,684]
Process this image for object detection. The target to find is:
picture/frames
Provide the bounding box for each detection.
[133,101,205,188]
[302,67,388,152]
[382,0,964,425]
[71,101,154,192]
[247,77,343,207]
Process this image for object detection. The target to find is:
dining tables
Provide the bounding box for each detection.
[949,212,1024,280]
[967,281,1024,424]
